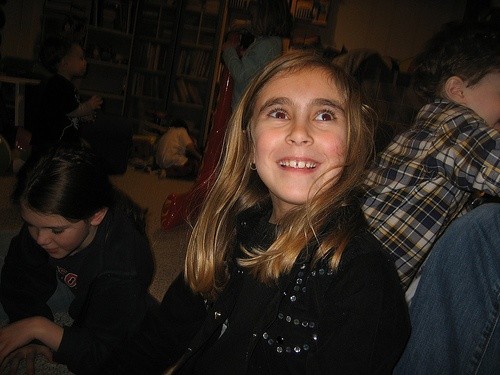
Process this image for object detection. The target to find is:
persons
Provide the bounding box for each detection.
[9,32,105,206]
[349,19,500,294]
[0,145,157,375]
[389,200,500,375]
[150,120,203,177]
[220,0,294,118]
[103,47,413,375]
[128,123,169,171]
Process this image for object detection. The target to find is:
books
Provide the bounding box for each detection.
[60,0,249,125]
[291,0,332,46]
